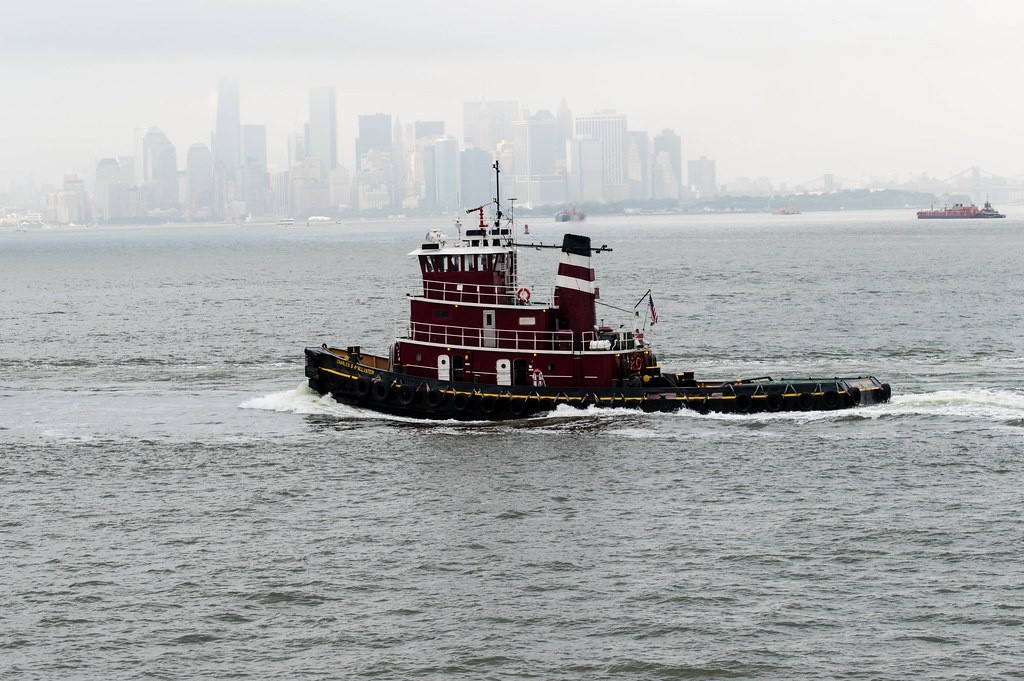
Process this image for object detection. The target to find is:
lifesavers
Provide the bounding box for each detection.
[515,286,532,302]
[537,397,556,414]
[764,392,785,412]
[878,383,892,402]
[849,387,862,406]
[821,390,841,410]
[733,393,753,413]
[354,376,370,396]
[688,398,707,412]
[531,369,545,387]
[567,398,585,409]
[796,391,816,411]
[479,395,498,414]
[371,381,389,402]
[451,393,470,412]
[623,400,639,409]
[509,396,527,417]
[595,400,613,409]
[426,388,443,407]
[396,383,416,406]
[635,357,643,370]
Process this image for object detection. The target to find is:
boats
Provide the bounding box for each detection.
[917,193,1006,219]
[772,206,802,215]
[277,217,295,228]
[303,160,893,422]
[336,219,342,224]
[554,204,587,222]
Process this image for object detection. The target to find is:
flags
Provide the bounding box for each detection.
[648,294,657,323]
